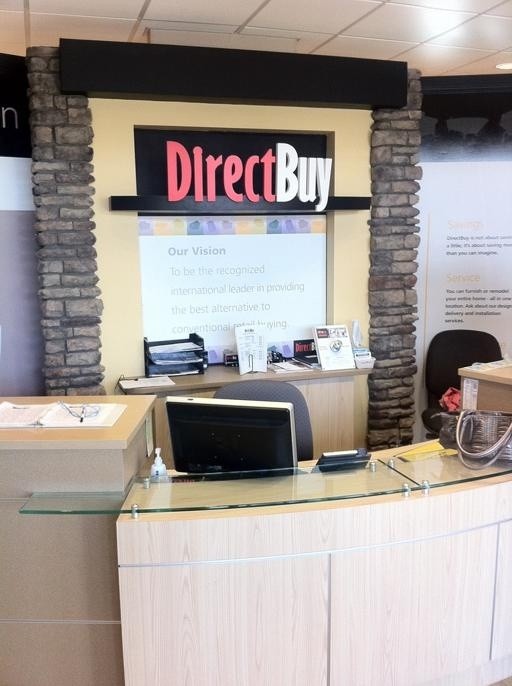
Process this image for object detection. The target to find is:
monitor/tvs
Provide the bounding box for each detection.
[165,395,298,473]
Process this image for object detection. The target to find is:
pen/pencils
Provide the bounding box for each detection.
[80,408,85,423]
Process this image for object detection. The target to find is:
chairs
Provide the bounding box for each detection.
[212,377,314,460]
[421,330,502,438]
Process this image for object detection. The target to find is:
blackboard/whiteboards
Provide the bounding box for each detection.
[317,447,371,472]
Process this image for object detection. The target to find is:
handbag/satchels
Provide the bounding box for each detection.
[430,410,512,470]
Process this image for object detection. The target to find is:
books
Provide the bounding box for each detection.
[267,354,322,374]
[147,363,200,378]
[145,350,204,366]
[311,323,355,370]
[148,342,204,354]
[312,448,373,475]
[0,400,128,428]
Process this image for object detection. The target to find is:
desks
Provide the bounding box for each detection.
[118,353,375,467]
